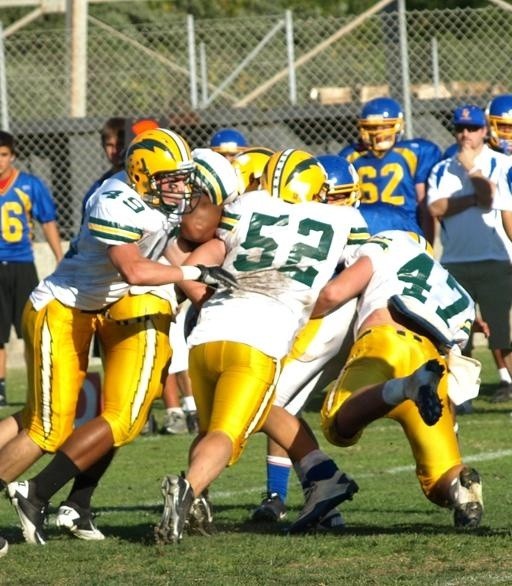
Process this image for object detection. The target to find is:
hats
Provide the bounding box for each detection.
[452,105,488,126]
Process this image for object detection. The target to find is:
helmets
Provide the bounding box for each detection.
[210,128,247,155]
[229,147,363,208]
[406,230,435,260]
[124,127,202,214]
[356,98,405,152]
[484,94,512,152]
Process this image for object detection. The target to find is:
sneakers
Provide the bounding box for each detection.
[6,477,51,545]
[453,466,483,529]
[252,458,360,532]
[488,380,512,402]
[160,410,188,435]
[414,359,445,426]
[54,499,106,542]
[152,470,217,546]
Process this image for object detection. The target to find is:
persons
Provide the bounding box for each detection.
[2,92,512,562]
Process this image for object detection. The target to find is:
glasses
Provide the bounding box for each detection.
[454,126,481,133]
